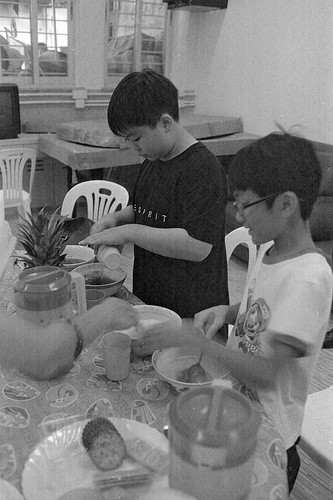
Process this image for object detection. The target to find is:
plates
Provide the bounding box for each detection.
[20,413,197,500]
[0,476,25,500]
[114,304,182,356]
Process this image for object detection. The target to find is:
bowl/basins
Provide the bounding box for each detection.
[70,261,126,298]
[59,244,96,272]
[151,338,236,390]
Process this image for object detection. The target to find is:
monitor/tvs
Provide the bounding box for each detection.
[0,82,20,139]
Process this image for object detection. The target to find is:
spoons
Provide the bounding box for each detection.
[187,310,216,384]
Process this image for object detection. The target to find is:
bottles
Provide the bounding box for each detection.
[170,382,260,499]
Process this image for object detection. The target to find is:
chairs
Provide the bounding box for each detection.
[298,385,333,475]
[0,147,37,235]
[222,226,274,348]
[62,180,129,222]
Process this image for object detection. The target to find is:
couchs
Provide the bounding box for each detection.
[220,138,333,267]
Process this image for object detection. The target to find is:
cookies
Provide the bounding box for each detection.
[82,418,150,489]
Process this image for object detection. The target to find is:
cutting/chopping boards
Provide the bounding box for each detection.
[56,110,242,147]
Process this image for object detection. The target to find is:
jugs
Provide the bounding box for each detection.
[11,267,76,380]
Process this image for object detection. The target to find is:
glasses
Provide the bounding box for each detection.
[232,191,284,215]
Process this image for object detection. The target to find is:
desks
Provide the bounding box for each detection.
[39,134,264,220]
[0,249,290,500]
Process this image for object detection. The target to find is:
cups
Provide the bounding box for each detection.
[77,288,105,308]
[95,244,122,270]
[100,333,131,381]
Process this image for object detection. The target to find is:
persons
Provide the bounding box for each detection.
[78,68,228,345]
[161,121,333,496]
